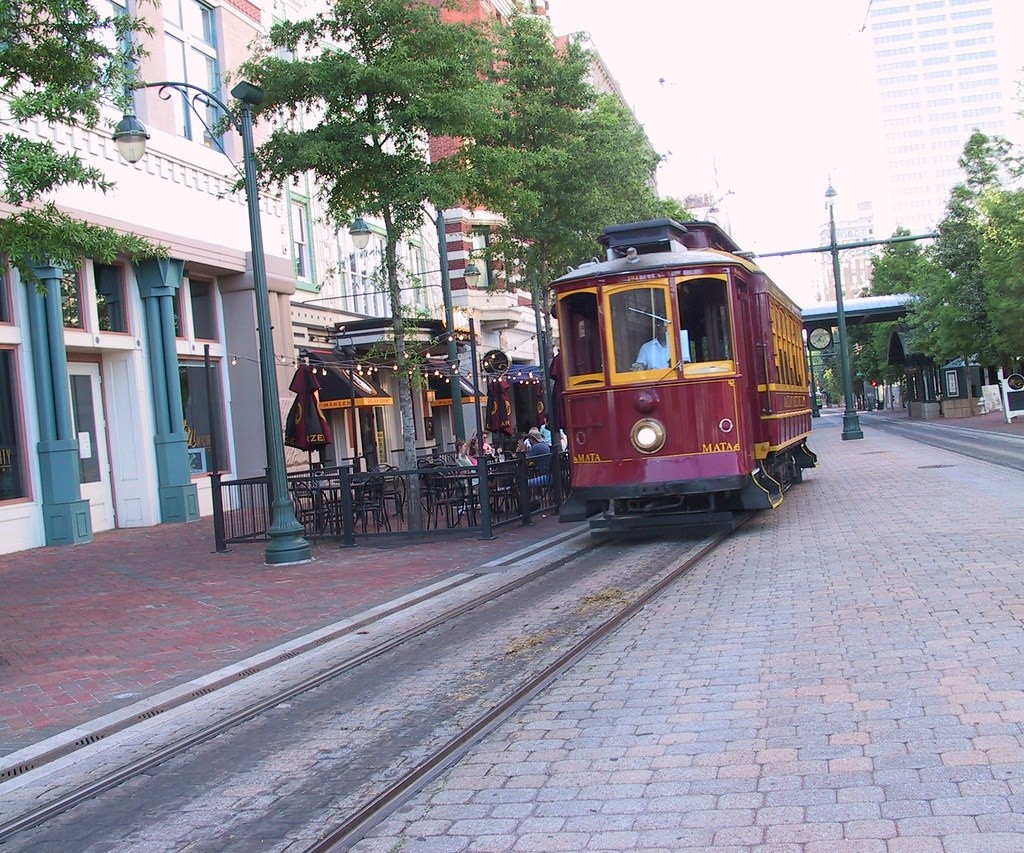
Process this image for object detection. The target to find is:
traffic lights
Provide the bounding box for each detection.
[870,380,878,386]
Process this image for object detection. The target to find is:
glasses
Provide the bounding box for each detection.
[484,436,487,439]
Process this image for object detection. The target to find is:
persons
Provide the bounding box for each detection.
[630,314,692,371]
[454,414,568,519]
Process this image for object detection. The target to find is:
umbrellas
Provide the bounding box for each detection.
[549,354,566,431]
[534,383,546,426]
[284,367,333,503]
[484,376,513,437]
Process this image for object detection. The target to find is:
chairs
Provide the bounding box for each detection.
[291,463,405,536]
[417,454,570,529]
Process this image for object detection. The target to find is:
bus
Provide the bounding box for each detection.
[546,217,818,535]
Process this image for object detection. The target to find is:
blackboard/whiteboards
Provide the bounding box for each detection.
[1002,374,1024,418]
[981,385,1003,410]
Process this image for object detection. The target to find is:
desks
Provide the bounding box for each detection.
[438,472,516,528]
[288,483,379,535]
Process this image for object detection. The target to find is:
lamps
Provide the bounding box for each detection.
[310,334,356,356]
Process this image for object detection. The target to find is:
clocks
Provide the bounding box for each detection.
[809,327,831,349]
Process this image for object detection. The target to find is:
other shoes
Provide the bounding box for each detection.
[529,500,540,511]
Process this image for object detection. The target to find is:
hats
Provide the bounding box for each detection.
[530,430,544,443]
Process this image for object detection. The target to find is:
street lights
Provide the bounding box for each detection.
[825,174,867,440]
[111,78,315,566]
[350,199,467,449]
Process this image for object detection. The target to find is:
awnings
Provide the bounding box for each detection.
[309,353,394,410]
[424,358,488,407]
